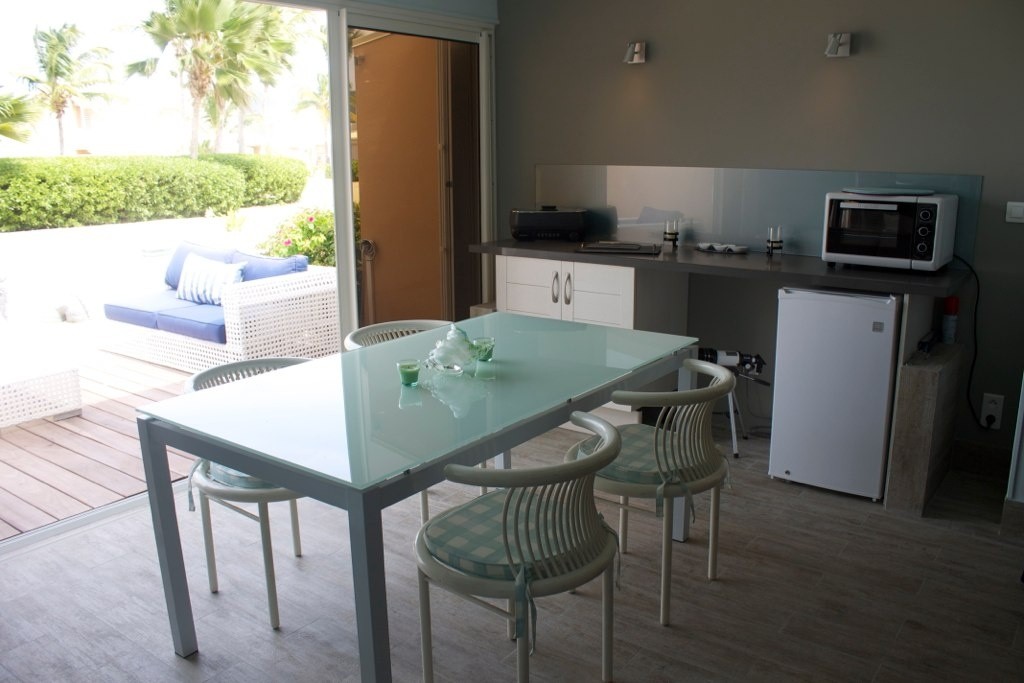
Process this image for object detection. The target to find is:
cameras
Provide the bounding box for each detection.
[693,348,766,374]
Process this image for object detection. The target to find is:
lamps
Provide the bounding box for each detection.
[621,39,646,66]
[824,30,852,60]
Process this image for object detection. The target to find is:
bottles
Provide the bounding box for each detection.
[942,310,957,344]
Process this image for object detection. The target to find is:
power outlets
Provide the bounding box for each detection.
[980,392,1007,431]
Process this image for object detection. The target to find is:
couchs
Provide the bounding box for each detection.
[0,287,84,429]
[91,240,336,372]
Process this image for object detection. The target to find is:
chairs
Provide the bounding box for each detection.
[180,354,330,634]
[558,358,745,628]
[411,407,624,683]
[344,314,489,520]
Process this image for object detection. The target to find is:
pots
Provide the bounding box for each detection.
[509,204,587,241]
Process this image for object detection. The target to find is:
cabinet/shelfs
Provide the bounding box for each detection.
[493,254,691,435]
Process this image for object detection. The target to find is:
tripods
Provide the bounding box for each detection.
[722,369,768,460]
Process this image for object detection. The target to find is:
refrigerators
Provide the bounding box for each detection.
[768,284,903,504]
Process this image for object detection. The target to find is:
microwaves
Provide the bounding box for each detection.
[822,186,959,276]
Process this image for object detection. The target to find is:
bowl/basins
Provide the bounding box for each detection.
[697,242,748,252]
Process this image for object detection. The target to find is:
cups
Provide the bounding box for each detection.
[767,224,782,247]
[397,359,421,386]
[665,220,678,239]
[473,336,495,362]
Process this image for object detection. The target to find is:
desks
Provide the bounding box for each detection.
[460,230,969,508]
[133,305,697,683]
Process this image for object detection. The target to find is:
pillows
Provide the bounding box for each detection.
[175,252,244,307]
